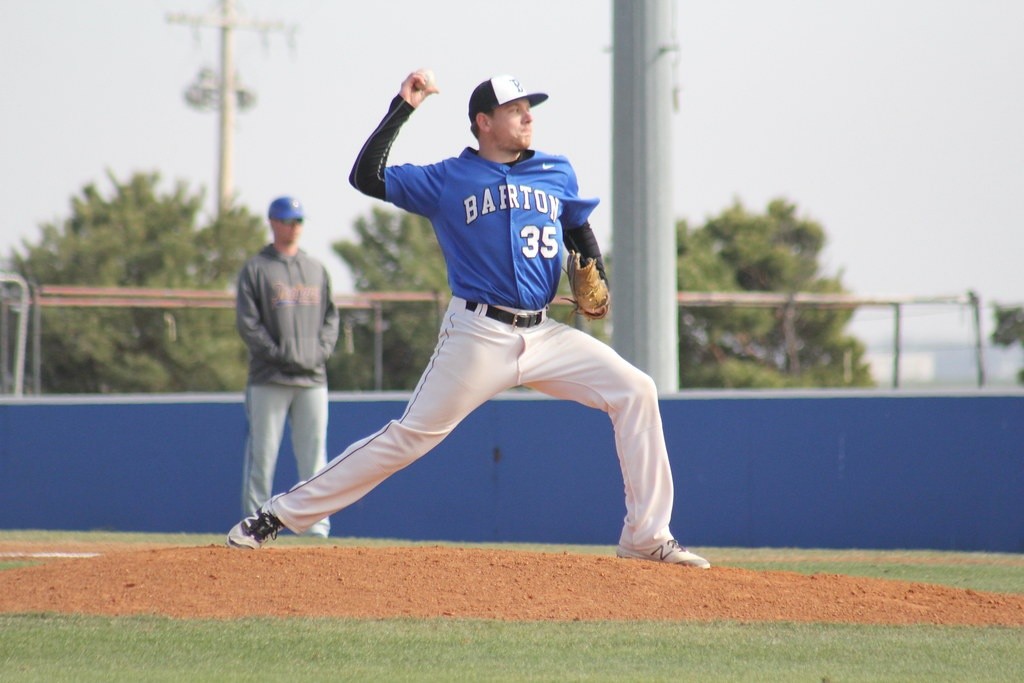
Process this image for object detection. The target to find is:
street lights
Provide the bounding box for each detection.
[181,67,261,284]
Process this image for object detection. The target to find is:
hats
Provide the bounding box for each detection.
[268,197,304,221]
[468,76,549,124]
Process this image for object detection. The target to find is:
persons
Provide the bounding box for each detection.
[235,197,341,536]
[221,67,710,569]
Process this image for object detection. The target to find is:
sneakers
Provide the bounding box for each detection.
[225,500,286,549]
[616,539,710,569]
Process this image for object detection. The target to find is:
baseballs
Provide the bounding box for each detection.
[414,70,434,89]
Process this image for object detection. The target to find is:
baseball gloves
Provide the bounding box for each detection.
[566,249,611,320]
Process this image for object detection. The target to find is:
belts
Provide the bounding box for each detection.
[466,300,542,329]
